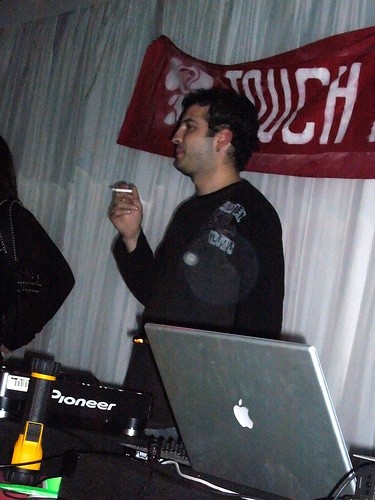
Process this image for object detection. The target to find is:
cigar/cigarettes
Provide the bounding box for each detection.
[111,188,132,193]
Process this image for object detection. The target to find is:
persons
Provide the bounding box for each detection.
[108,86,285,430]
[0,135,75,360]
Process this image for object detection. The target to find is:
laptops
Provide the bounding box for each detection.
[143,323,357,500]
